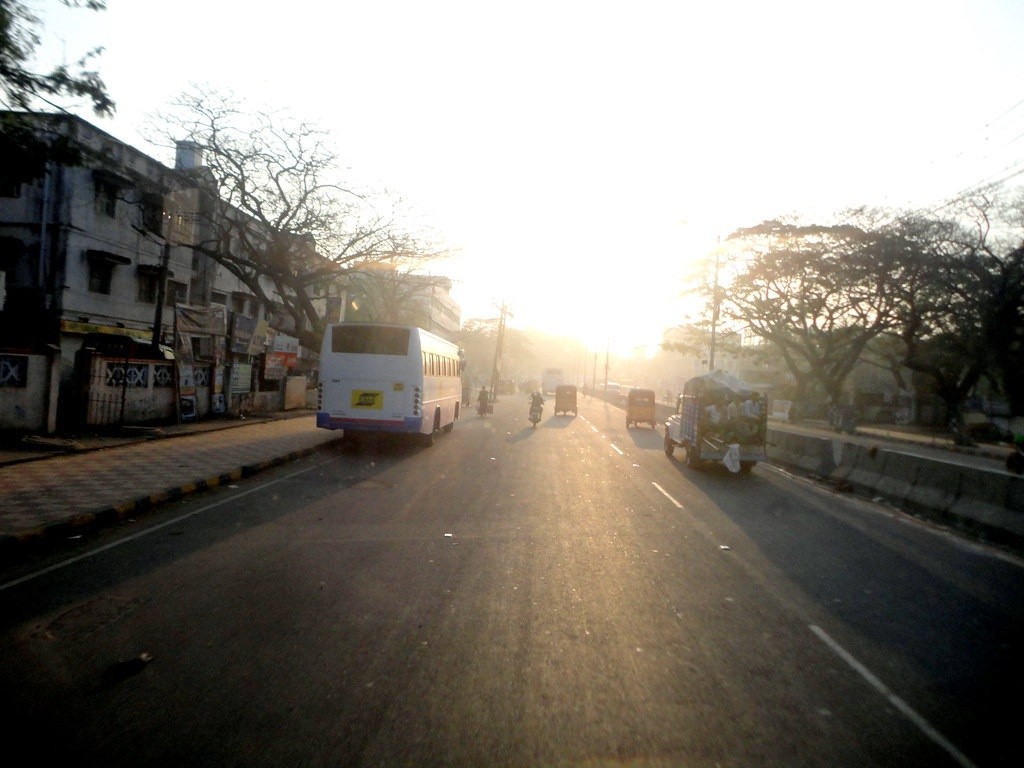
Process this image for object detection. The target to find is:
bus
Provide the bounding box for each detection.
[316,322,463,448]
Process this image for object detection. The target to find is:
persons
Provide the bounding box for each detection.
[702,390,763,444]
[478,387,492,417]
[528,392,544,422]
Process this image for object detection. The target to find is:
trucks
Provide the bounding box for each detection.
[665,392,770,474]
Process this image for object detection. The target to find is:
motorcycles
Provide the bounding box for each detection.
[554,385,578,417]
[626,387,657,430]
[530,398,542,427]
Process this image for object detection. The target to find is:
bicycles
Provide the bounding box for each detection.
[478,398,487,417]
